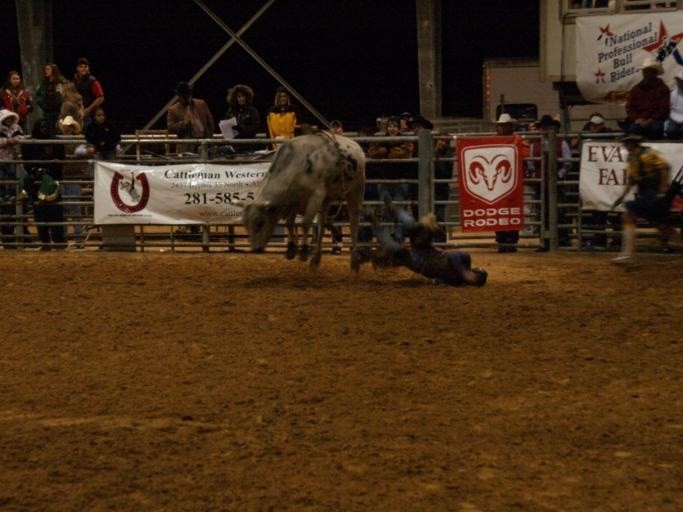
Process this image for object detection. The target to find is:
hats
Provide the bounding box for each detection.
[492,111,518,125]
[533,115,560,129]
[589,114,604,126]
[173,82,193,96]
[54,115,81,134]
[614,132,643,146]
[78,58,88,65]
[226,84,254,108]
[617,117,632,130]
[635,57,665,75]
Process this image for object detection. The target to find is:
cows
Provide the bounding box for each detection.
[229,132,367,281]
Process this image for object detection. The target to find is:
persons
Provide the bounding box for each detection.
[363,191,487,285]
[150,81,454,253]
[491,58,683,253]
[607,131,674,264]
[1,58,120,253]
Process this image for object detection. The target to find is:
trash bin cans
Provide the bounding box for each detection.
[496,103,538,135]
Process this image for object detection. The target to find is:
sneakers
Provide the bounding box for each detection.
[495,245,547,253]
[609,256,636,266]
